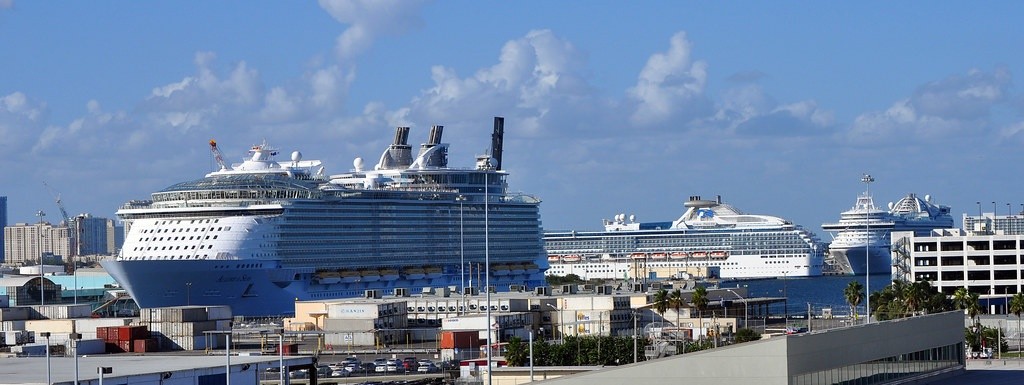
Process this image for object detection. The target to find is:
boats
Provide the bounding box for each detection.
[313,271,342,285]
[425,266,442,280]
[523,263,540,276]
[691,252,708,260]
[710,251,727,260]
[630,253,646,261]
[546,254,560,263]
[339,270,361,284]
[379,268,400,282]
[669,251,687,261]
[563,254,581,263]
[360,270,380,283]
[651,252,667,261]
[509,264,525,276]
[491,265,511,277]
[403,268,426,281]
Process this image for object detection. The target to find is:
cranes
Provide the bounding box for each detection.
[43,181,70,225]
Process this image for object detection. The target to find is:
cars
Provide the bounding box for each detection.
[964,346,994,360]
[264,355,459,385]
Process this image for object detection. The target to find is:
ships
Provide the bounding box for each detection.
[540,195,856,282]
[820,191,955,276]
[98,117,550,323]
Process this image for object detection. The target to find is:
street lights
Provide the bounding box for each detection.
[474,150,495,385]
[1020,203,1024,235]
[1007,203,1011,235]
[782,271,789,318]
[40,332,52,385]
[96,366,113,385]
[727,289,748,329]
[455,194,467,316]
[988,288,995,315]
[859,173,874,325]
[36,210,47,306]
[992,202,996,235]
[185,282,192,306]
[977,201,981,234]
[1006,287,1010,315]
